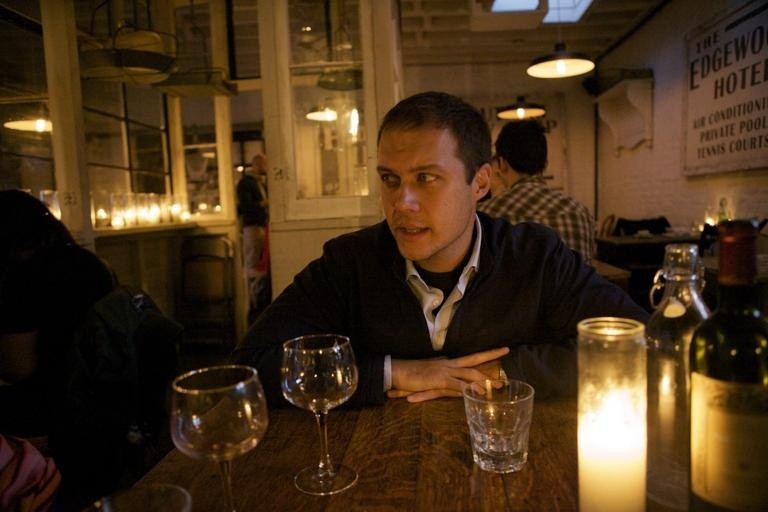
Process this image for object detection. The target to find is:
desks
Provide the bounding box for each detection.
[103,392,768,511]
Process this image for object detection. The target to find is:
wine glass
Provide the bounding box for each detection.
[280,331,364,497]
[170,362,270,512]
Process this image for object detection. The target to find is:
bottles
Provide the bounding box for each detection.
[684,218,768,511]
[643,242,714,512]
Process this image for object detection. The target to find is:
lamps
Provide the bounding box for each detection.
[526,1,595,79]
[496,95,547,120]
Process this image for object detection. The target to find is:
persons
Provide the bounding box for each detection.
[0,189,202,512]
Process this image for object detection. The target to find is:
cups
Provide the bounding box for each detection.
[463,377,535,478]
